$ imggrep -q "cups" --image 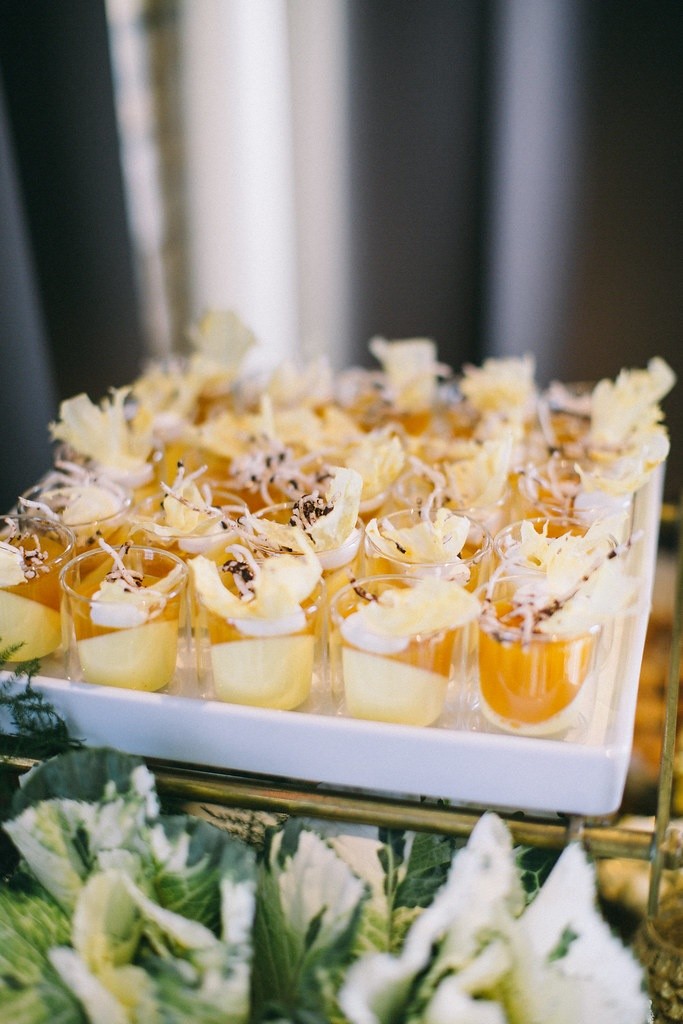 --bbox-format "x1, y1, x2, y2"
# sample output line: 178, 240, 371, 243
0, 361, 671, 738
